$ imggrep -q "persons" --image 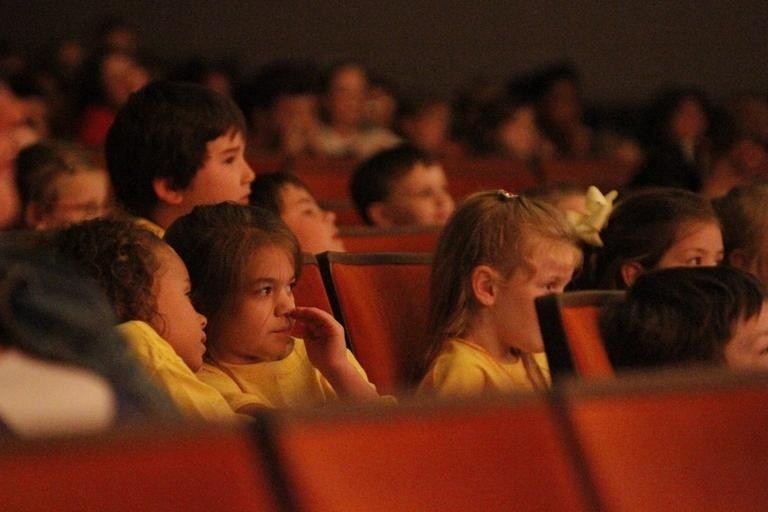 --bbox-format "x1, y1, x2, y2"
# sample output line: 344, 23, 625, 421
3, 21, 767, 443
